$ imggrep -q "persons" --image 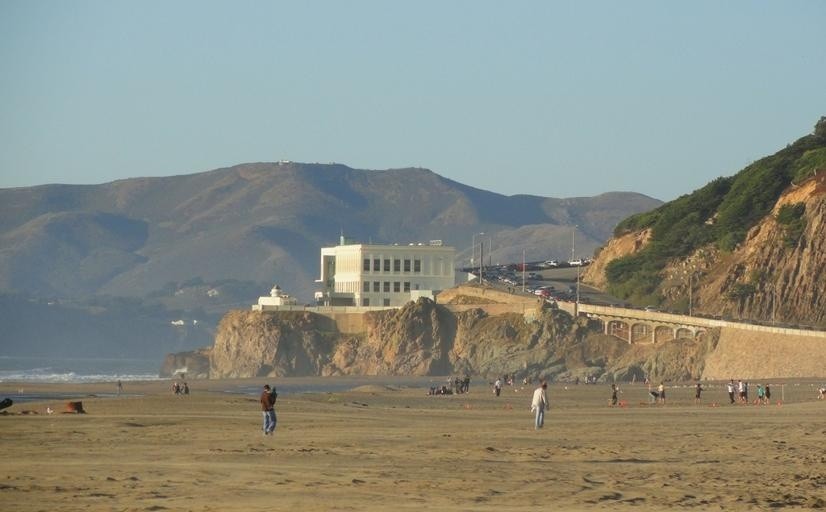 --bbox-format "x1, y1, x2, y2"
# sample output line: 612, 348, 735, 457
529, 380, 550, 430
737, 379, 744, 404
611, 383, 619, 405
695, 383, 704, 407
764, 382, 771, 405
756, 383, 766, 408
172, 381, 181, 395
657, 381, 667, 405
647, 382, 658, 403
115, 379, 124, 398
727, 378, 736, 406
816, 387, 826, 400
265, 384, 278, 406
421, 371, 597, 398
743, 381, 750, 405
260, 384, 276, 434
181, 382, 189, 394
